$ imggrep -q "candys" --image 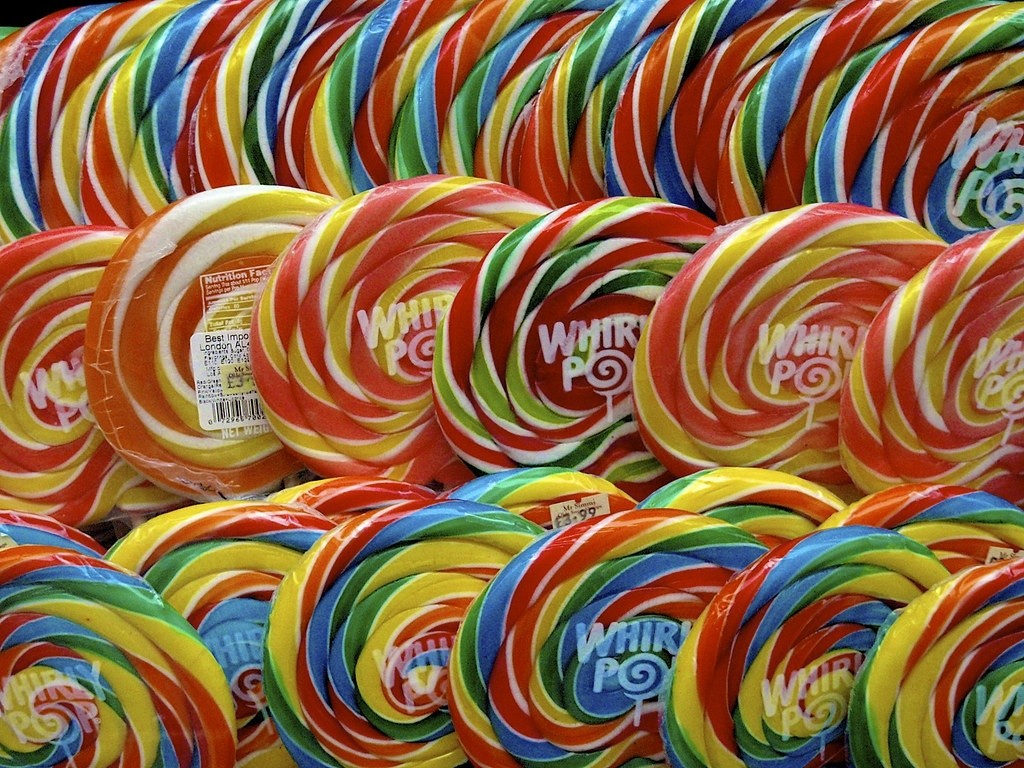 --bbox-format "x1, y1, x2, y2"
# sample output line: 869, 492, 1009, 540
0, 0, 1023, 768
